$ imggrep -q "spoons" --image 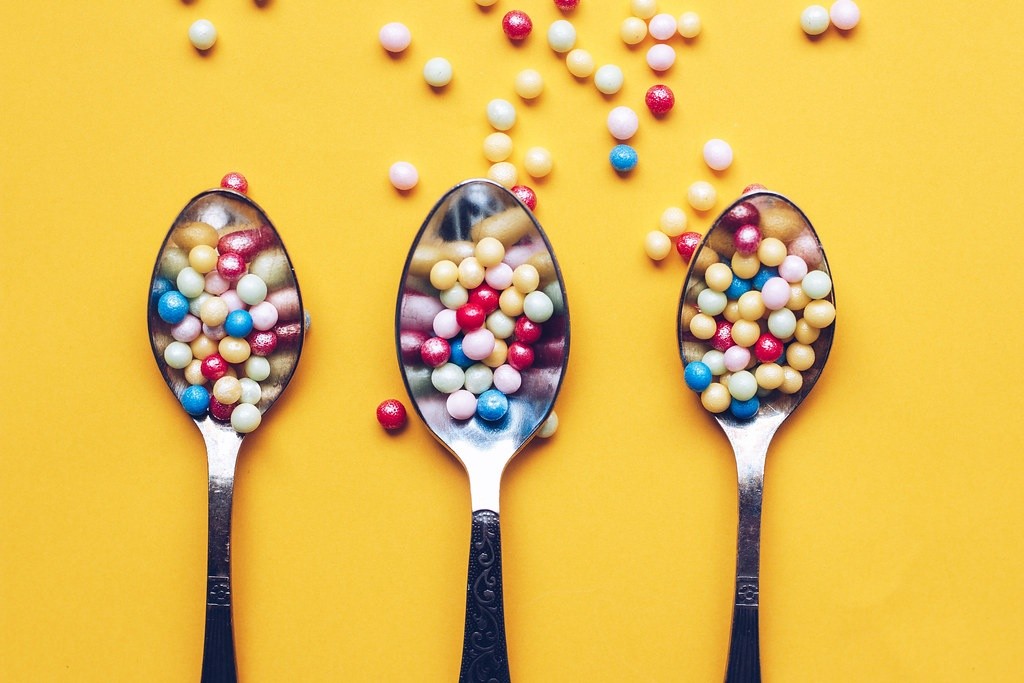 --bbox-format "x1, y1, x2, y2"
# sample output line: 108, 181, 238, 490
148, 188, 304, 683
394, 180, 569, 683
676, 193, 835, 683
